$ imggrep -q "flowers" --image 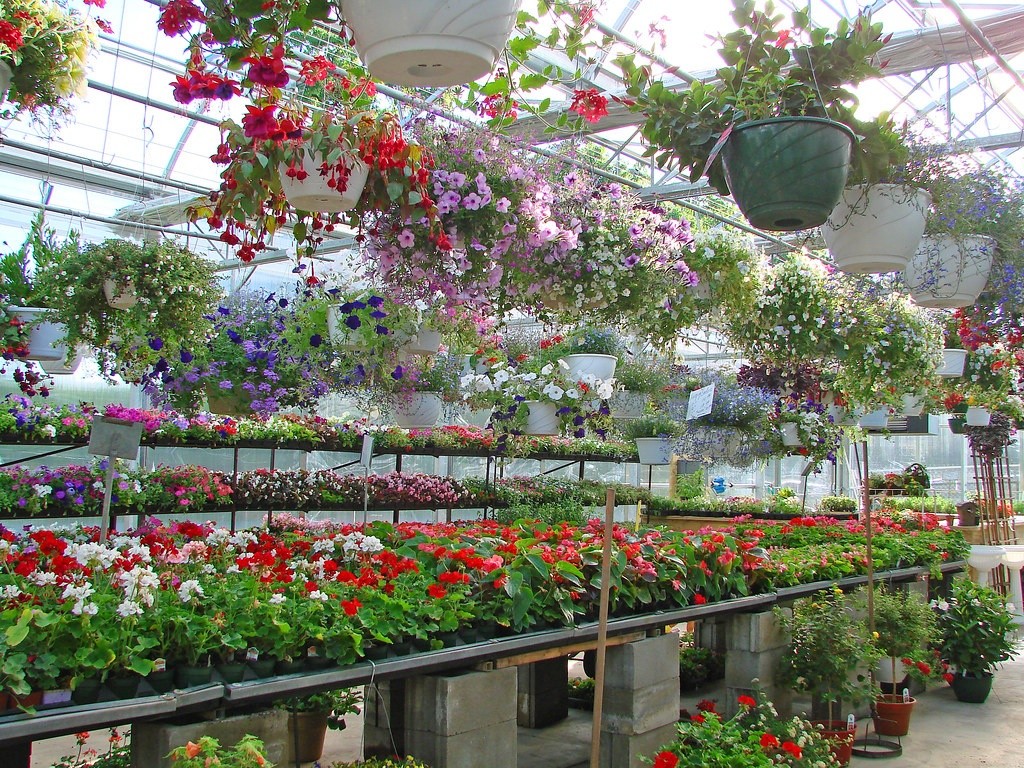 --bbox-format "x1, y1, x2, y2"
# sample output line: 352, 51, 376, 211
0, 1, 1023, 767
